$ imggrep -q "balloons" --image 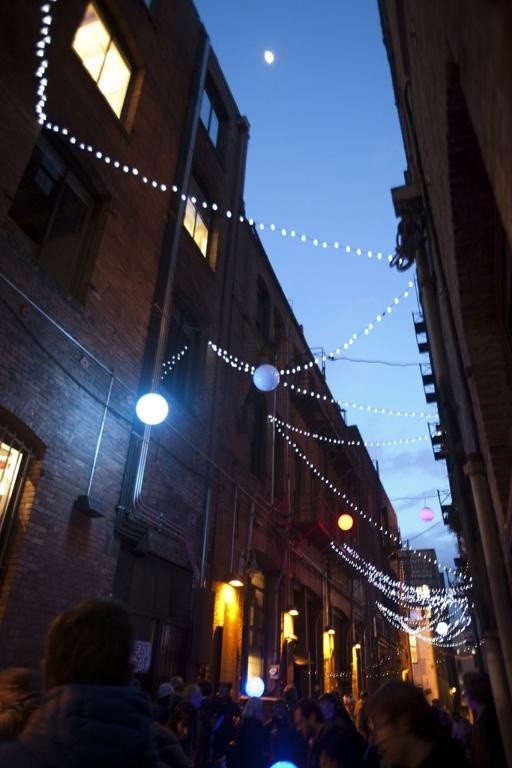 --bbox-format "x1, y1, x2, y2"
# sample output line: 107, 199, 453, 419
437, 622, 448, 635
254, 364, 280, 393
418, 506, 434, 522
